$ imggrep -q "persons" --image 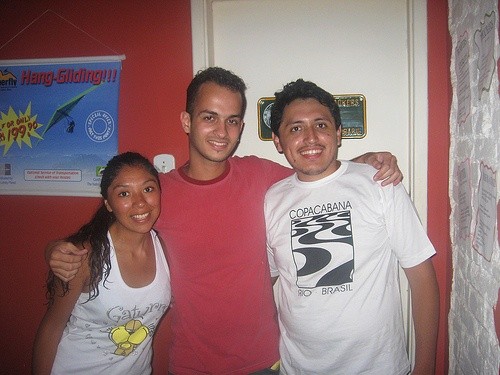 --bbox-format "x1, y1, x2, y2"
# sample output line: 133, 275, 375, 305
31, 152, 172, 375
43, 67, 403, 375
263, 79, 440, 375
